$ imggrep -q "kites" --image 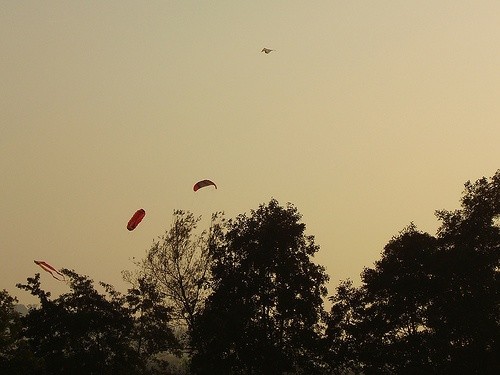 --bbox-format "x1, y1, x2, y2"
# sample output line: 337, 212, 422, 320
35, 261, 66, 281
